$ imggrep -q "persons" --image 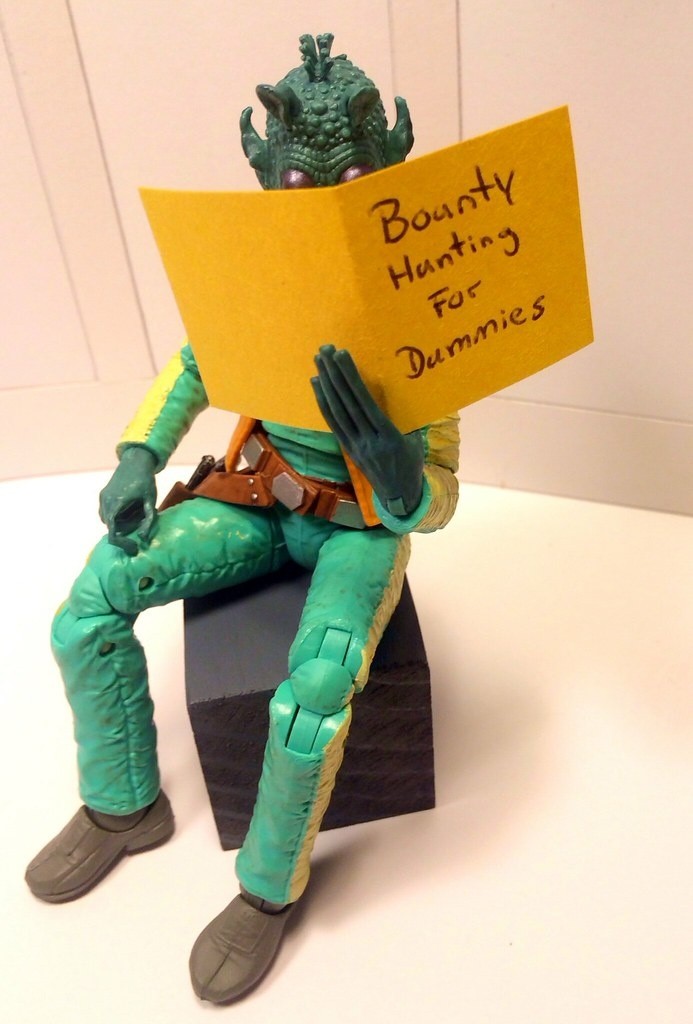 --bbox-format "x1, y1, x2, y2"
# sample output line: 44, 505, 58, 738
27, 33, 461, 1003
